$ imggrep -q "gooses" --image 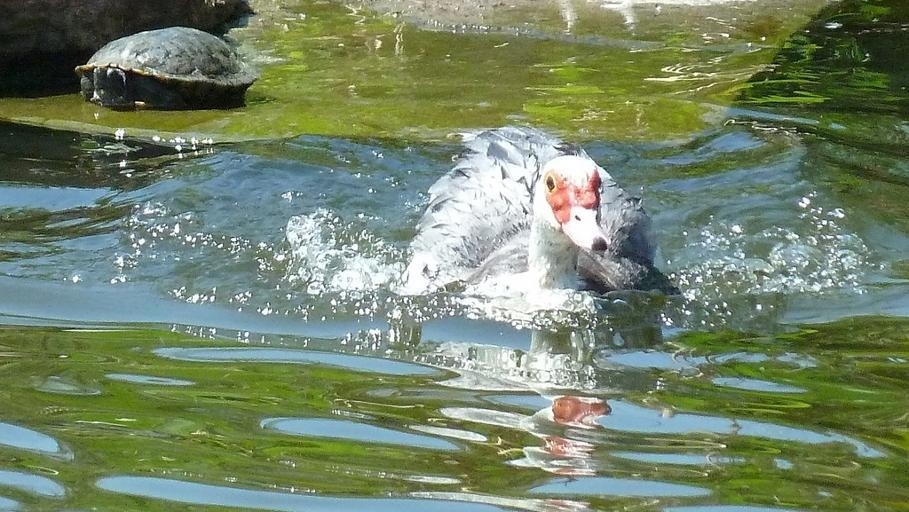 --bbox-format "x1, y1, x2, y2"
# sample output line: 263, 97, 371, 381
390, 122, 683, 332
